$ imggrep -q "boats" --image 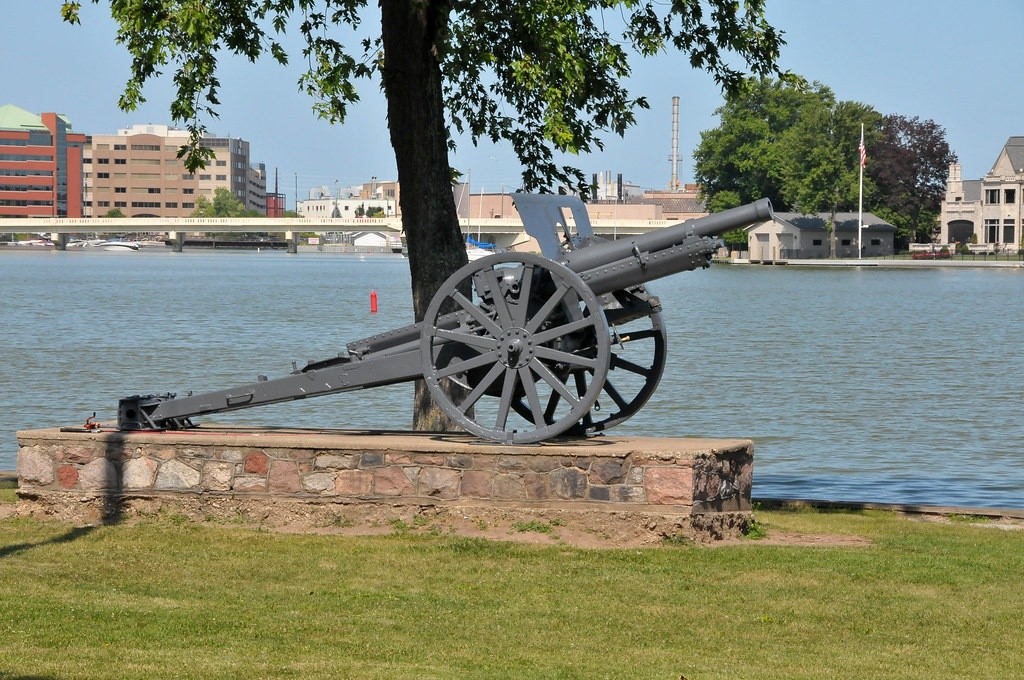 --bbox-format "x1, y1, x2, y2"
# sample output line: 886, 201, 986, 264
1, 235, 140, 254
